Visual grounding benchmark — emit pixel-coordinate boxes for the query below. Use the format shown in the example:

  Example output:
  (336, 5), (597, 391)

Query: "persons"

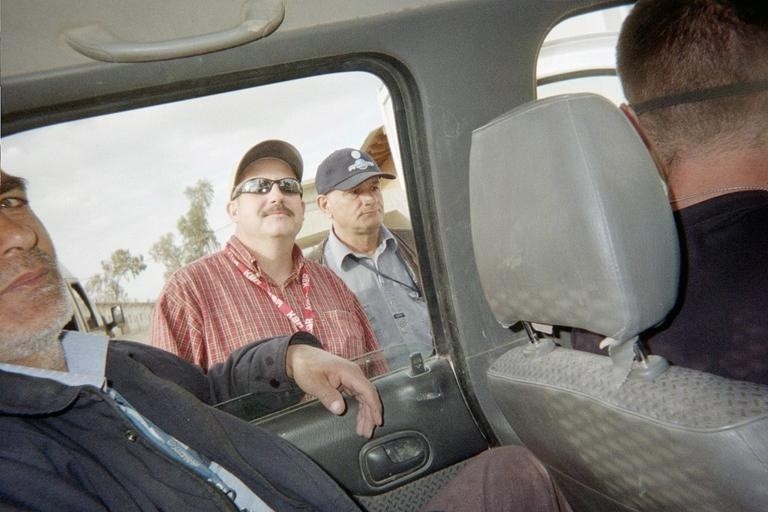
(145, 137), (392, 407)
(0, 171), (570, 512)
(305, 147), (437, 372)
(551, 2), (768, 386)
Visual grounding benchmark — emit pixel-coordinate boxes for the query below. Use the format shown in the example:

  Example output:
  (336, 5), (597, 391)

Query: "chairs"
(467, 92), (768, 503)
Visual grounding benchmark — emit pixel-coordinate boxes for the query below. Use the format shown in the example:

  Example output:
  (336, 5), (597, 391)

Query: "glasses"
(232, 176), (304, 202)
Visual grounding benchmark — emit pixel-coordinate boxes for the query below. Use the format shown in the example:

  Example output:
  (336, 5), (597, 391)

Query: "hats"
(315, 148), (396, 194)
(229, 138), (304, 188)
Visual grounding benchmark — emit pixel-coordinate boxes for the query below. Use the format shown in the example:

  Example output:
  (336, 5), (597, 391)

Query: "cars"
(57, 262), (126, 339)
